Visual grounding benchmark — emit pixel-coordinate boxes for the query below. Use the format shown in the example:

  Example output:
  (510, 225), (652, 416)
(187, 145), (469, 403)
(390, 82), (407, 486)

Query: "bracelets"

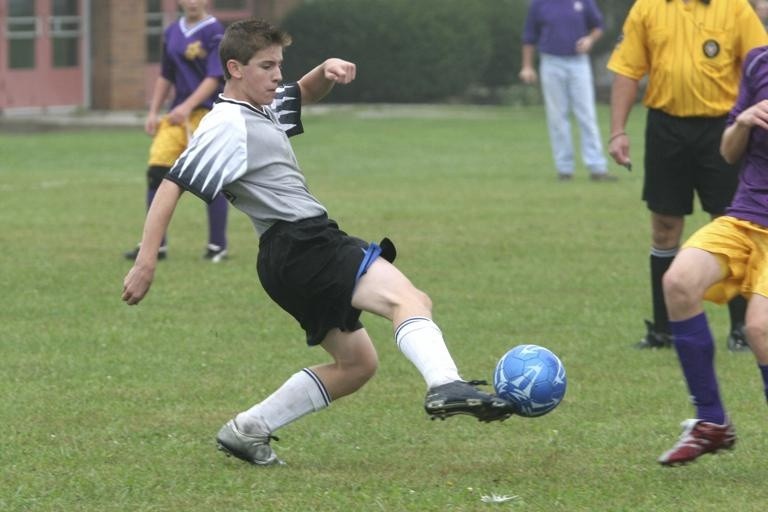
(609, 130), (629, 146)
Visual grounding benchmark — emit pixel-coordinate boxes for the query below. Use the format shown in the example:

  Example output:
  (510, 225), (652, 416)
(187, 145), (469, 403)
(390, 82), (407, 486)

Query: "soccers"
(495, 345), (567, 417)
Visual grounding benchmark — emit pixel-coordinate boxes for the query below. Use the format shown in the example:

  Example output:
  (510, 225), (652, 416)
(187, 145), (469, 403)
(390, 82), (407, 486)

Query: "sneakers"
(215, 421), (284, 466)
(424, 379), (512, 424)
(635, 314), (676, 349)
(724, 327), (746, 349)
(126, 242), (167, 260)
(657, 414), (736, 466)
(205, 244), (227, 260)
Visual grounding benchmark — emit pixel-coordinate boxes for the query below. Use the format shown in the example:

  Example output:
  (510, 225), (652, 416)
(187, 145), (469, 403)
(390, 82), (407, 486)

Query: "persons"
(123, 0), (230, 267)
(517, 0), (615, 181)
(117, 18), (517, 465)
(607, 0), (767, 355)
(655, 44), (768, 467)
